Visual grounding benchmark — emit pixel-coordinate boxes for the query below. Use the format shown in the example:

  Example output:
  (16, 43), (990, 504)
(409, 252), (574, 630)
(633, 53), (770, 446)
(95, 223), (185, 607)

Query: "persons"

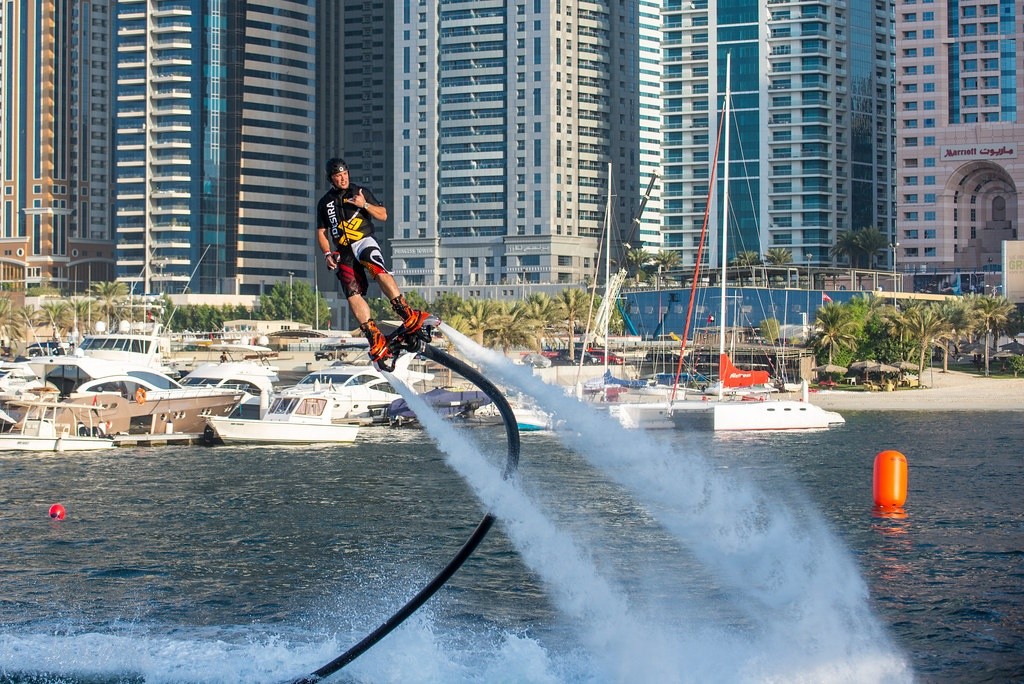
(220, 351), (227, 362)
(317, 157), (417, 357)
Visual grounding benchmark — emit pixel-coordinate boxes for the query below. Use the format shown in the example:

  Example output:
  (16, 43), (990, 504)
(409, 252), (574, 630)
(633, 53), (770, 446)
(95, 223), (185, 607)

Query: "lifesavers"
(135, 387), (147, 404)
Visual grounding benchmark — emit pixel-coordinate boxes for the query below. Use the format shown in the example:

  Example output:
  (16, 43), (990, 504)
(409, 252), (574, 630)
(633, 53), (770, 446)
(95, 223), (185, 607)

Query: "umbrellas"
(998, 339), (1024, 354)
(993, 350), (1019, 363)
(892, 361), (919, 378)
(865, 362), (899, 381)
(850, 359), (877, 381)
(811, 363), (848, 382)
(959, 339), (997, 362)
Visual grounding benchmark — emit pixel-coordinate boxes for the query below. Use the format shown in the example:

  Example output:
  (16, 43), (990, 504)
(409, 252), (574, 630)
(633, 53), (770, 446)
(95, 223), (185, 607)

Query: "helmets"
(326, 158), (349, 184)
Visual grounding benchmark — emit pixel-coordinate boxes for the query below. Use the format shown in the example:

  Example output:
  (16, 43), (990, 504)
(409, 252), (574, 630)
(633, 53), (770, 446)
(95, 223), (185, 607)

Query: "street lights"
(889, 242), (900, 308)
(805, 254), (813, 325)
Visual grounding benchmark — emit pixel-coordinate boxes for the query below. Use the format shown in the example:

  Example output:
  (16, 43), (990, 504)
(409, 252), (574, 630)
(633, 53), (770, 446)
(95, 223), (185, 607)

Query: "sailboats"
(473, 53), (846, 434)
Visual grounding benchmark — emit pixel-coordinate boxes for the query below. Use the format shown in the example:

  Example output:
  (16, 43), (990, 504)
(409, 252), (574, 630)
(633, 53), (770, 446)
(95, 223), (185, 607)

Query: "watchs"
(363, 202), (370, 208)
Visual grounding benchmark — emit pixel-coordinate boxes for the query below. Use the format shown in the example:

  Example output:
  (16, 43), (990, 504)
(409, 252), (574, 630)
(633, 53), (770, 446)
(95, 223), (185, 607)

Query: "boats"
(198, 392), (360, 445)
(0, 400), (116, 452)
(0, 242), (435, 445)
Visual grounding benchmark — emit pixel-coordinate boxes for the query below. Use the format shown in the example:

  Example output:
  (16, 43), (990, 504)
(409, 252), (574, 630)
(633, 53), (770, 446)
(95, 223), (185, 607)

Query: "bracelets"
(323, 249), (332, 259)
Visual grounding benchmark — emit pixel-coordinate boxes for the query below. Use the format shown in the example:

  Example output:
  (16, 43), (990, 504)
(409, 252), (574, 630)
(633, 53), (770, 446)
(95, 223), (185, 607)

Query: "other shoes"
(360, 319), (386, 356)
(390, 294), (420, 329)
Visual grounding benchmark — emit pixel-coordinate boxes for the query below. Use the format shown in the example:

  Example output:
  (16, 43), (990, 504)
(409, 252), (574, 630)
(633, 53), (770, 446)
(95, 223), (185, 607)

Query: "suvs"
(314, 344), (349, 362)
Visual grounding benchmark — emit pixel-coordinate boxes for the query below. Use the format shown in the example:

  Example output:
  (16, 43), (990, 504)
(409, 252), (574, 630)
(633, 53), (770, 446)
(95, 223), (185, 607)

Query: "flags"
(822, 291), (832, 302)
